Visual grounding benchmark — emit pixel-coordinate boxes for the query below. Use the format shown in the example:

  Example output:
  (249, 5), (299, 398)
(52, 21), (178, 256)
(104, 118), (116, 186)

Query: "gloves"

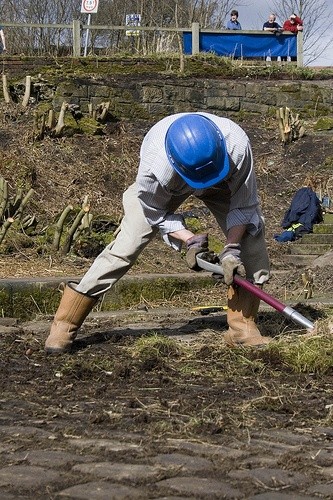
(217, 243), (247, 286)
(185, 233), (219, 271)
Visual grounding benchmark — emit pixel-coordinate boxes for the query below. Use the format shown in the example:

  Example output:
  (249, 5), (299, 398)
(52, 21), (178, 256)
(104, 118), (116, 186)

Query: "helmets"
(165, 114), (230, 189)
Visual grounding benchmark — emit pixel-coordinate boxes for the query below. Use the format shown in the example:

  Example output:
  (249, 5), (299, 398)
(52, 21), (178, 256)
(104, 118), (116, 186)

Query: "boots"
(45, 281), (100, 351)
(225, 283), (276, 348)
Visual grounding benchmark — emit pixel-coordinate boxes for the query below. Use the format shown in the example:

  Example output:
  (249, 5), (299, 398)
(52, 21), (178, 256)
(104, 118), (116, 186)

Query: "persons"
(45, 111), (276, 354)
(262, 14), (285, 31)
(283, 14), (304, 32)
(226, 10), (242, 30)
